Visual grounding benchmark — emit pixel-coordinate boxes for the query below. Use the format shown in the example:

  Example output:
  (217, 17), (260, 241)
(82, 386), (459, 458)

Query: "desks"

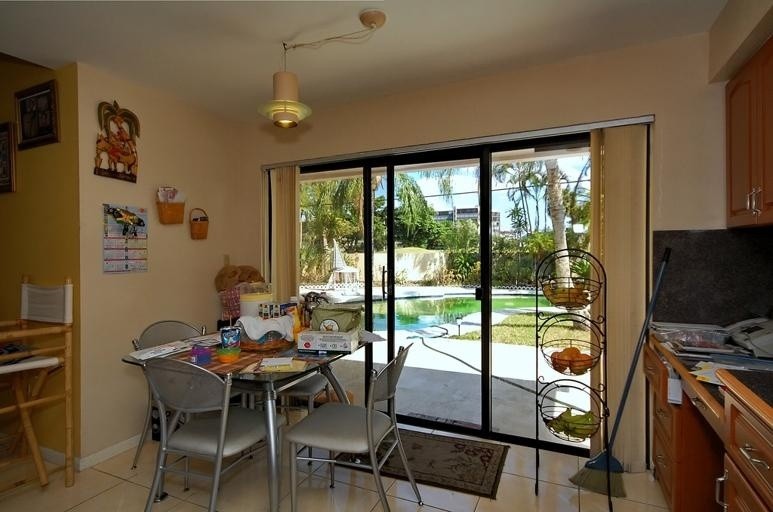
(643, 334), (773, 511)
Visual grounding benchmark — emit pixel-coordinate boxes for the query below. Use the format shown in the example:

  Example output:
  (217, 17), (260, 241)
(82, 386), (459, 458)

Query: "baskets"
(237, 323), (293, 351)
(540, 318), (601, 376)
(540, 385), (600, 443)
(540, 255), (602, 309)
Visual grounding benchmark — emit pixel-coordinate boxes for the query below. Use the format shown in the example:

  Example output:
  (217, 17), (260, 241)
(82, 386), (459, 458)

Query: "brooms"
(566, 248), (677, 498)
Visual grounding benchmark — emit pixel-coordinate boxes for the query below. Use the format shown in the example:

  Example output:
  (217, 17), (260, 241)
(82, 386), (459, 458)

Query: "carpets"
(328, 427), (511, 500)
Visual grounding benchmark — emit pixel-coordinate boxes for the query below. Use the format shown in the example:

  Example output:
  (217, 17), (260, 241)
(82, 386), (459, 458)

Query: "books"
(664, 337), (752, 386)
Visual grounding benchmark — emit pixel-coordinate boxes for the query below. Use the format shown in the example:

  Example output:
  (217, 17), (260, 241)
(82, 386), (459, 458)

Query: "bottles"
(258, 296), (297, 320)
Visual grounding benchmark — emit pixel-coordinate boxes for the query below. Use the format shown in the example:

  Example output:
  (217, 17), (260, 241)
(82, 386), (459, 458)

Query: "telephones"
(726, 318), (773, 350)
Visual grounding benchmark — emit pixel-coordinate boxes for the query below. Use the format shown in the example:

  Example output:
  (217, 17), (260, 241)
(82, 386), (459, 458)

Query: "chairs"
(284, 342), (424, 511)
(130, 320), (332, 511)
(0, 274), (75, 489)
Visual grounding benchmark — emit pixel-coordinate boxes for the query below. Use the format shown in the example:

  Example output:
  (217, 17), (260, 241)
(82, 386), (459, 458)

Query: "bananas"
(544, 280), (589, 307)
(547, 407), (594, 438)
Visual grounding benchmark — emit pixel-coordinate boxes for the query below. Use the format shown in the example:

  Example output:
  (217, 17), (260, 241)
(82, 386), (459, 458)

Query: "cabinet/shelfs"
(725, 35), (773, 230)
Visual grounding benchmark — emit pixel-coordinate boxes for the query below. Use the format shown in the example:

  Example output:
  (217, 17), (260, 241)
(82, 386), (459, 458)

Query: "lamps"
(256, 8), (386, 130)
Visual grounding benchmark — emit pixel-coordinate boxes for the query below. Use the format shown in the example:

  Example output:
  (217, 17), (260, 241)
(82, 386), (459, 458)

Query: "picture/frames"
(14, 78), (62, 152)
(0, 121), (16, 193)
